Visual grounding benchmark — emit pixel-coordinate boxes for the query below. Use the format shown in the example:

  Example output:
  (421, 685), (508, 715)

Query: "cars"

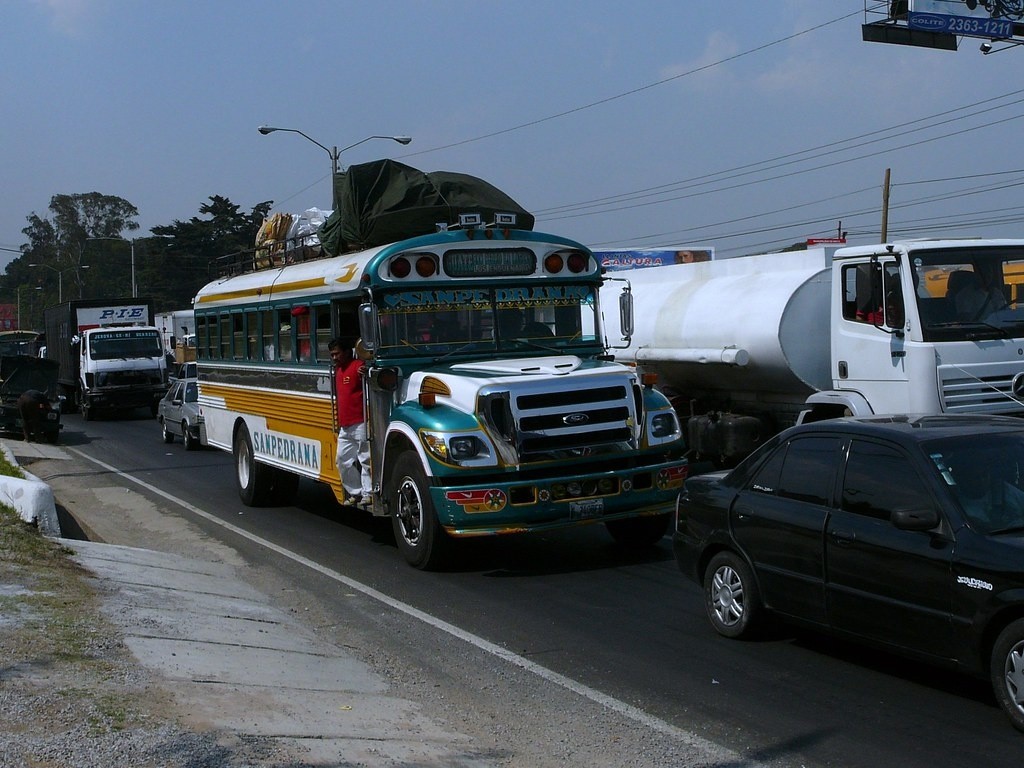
(675, 415), (1024, 724)
(158, 379), (199, 448)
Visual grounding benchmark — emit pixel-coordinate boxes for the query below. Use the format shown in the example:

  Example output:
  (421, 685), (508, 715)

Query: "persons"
(17, 389), (51, 443)
(857, 268), (919, 328)
(956, 260), (1009, 322)
(327, 337), (375, 506)
(82, 341), (99, 354)
(677, 250), (695, 263)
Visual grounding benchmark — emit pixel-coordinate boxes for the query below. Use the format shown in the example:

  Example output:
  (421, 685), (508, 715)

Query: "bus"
(0, 330), (40, 357)
(194, 229), (688, 568)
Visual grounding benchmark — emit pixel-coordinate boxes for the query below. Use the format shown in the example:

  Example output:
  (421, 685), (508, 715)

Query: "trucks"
(43, 298), (167, 420)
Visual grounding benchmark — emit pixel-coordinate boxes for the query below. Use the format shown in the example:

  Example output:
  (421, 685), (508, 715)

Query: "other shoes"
(344, 495), (363, 505)
(359, 497), (372, 506)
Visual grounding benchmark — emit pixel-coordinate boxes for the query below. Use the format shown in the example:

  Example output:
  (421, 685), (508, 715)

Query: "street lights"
(86, 235), (175, 298)
(258, 125), (412, 209)
(29, 264), (90, 303)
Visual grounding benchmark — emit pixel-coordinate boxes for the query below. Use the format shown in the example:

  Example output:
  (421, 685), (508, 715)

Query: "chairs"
(221, 320), (232, 359)
(263, 334), (275, 361)
(946, 271), (1007, 324)
(279, 330), (293, 361)
(316, 329), (332, 360)
(248, 335), (260, 361)
(234, 332), (246, 361)
(209, 324), (220, 358)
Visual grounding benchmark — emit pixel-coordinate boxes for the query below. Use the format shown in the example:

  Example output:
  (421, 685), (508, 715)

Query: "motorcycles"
(41, 395), (66, 444)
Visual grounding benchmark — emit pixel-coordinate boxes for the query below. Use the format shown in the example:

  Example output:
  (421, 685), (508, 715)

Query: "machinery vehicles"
(551, 239), (1024, 474)
